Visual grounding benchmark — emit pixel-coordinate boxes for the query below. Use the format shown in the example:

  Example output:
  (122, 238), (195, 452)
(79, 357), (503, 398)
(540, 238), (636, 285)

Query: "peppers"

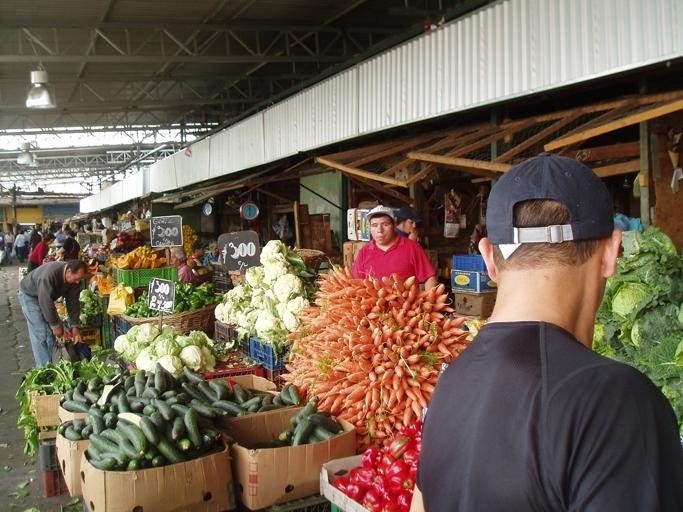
(122, 281), (222, 317)
(332, 420), (423, 512)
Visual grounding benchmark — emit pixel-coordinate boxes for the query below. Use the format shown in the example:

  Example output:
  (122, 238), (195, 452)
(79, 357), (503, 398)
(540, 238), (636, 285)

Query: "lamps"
(24, 59), (57, 110)
(16, 139), (41, 169)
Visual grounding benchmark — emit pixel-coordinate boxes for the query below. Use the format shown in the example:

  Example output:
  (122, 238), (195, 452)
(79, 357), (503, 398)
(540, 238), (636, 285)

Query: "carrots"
(276, 255), (472, 453)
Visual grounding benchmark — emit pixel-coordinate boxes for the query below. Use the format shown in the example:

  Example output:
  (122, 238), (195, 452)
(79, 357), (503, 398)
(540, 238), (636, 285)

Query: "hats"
(191, 240), (209, 251)
(396, 206), (423, 223)
(366, 205), (395, 223)
(485, 152), (614, 260)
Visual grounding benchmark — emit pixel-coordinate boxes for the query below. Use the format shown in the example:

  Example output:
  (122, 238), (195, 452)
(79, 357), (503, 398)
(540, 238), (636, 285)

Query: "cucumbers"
(59, 362), (343, 471)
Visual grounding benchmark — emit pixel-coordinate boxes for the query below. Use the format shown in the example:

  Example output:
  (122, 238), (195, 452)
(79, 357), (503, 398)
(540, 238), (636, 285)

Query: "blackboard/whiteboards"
(218, 230), (260, 271)
(148, 278), (175, 314)
(149, 214), (183, 247)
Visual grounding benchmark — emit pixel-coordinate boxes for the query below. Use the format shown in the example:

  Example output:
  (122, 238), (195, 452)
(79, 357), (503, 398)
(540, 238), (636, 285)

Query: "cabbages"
(79, 289), (101, 323)
(591, 222), (683, 436)
(113, 322), (217, 380)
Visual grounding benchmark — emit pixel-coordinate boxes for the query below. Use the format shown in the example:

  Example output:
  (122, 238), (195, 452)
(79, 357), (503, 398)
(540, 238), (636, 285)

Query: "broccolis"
(213, 239), (310, 343)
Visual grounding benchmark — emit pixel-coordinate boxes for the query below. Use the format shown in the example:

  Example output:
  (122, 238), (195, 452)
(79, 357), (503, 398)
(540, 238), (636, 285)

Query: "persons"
(185, 240), (209, 269)
(0, 217), (105, 263)
(349, 204), (438, 291)
(394, 206), (420, 238)
(170, 248), (194, 283)
(17, 260), (88, 368)
(407, 149), (682, 512)
(203, 240), (220, 267)
(27, 233), (56, 272)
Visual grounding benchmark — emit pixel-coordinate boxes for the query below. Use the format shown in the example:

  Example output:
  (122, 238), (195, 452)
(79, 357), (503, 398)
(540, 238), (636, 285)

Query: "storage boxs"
(34, 392), (66, 438)
(39, 440), (72, 498)
(211, 373), (275, 403)
(78, 431), (234, 510)
(53, 415), (90, 499)
(234, 388), (300, 420)
(56, 400), (90, 424)
(450, 268), (497, 321)
(234, 405), (357, 511)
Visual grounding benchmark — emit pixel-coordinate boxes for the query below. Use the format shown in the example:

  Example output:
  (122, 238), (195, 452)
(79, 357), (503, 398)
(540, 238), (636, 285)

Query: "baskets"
(112, 263), (178, 288)
(38, 438), (70, 498)
(292, 249), (325, 278)
(114, 302), (220, 339)
(452, 254), (488, 273)
(210, 264), (234, 297)
(264, 492), (345, 512)
(192, 320), (290, 392)
(63, 324), (101, 346)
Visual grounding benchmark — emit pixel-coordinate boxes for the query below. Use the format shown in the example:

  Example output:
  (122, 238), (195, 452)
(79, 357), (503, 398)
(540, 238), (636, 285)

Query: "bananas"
(116, 246), (163, 269)
